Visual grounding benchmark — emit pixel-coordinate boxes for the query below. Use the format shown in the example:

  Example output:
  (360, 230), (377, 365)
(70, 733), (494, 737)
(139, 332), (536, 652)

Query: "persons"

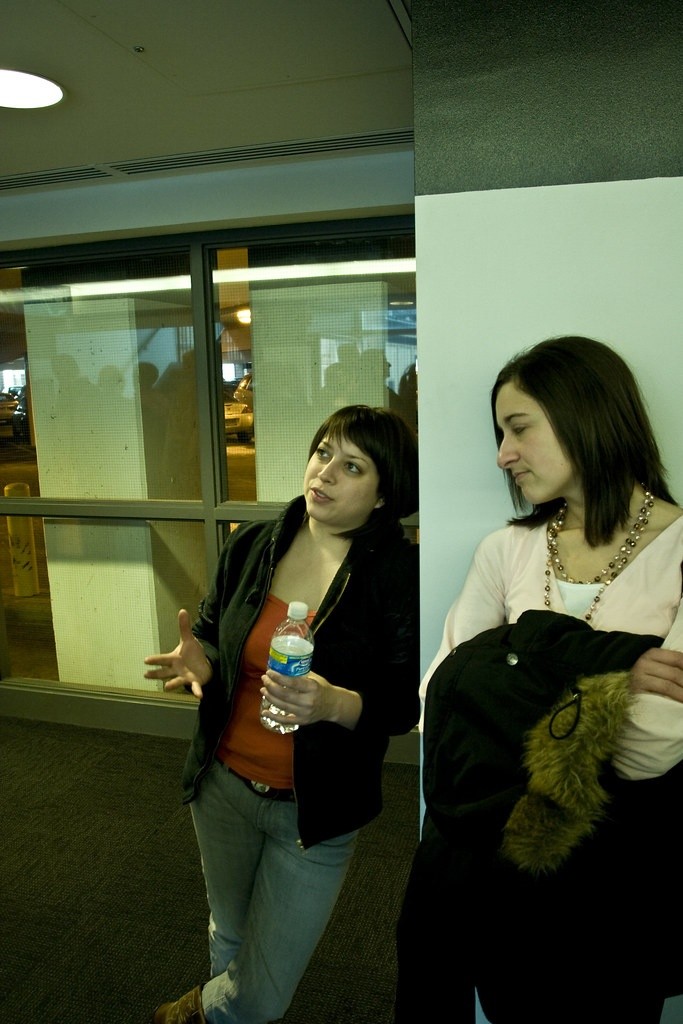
(37, 349), (198, 488)
(143, 405), (420, 1024)
(393, 336), (683, 1024)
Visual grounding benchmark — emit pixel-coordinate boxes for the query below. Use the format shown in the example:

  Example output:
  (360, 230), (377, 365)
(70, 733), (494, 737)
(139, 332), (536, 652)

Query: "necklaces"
(544, 483), (654, 620)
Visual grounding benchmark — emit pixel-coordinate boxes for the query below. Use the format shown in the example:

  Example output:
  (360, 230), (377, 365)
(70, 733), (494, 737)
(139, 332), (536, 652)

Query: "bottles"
(259, 600), (316, 735)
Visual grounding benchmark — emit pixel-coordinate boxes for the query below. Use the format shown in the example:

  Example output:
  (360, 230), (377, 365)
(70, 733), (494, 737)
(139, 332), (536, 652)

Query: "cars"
(10, 385), (29, 442)
(233, 375), (253, 411)
(9, 386), (23, 397)
(224, 392), (254, 443)
(0, 393), (19, 424)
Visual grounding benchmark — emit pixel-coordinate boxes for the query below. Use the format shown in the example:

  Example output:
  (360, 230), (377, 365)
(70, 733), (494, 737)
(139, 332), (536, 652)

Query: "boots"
(155, 983), (206, 1024)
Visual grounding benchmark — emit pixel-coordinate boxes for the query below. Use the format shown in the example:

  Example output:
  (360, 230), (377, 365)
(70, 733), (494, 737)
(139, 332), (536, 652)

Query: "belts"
(214, 753), (296, 803)
